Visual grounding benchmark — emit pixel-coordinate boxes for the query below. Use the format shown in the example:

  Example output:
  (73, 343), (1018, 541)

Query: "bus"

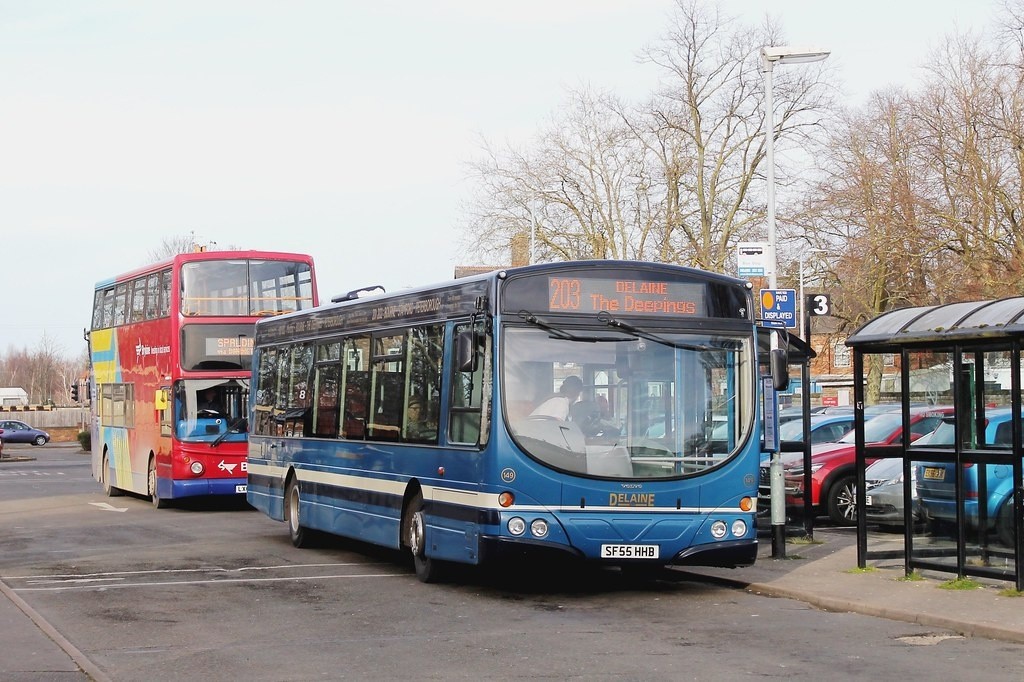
(245, 258), (789, 585)
(83, 252), (320, 510)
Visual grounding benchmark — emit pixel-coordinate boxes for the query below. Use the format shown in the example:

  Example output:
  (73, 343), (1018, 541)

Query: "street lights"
(798, 248), (830, 418)
(530, 189), (559, 264)
(760, 44), (831, 563)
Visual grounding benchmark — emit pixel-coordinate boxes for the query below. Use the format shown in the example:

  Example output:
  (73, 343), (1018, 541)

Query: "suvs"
(759, 405), (955, 527)
(916, 404), (1024, 546)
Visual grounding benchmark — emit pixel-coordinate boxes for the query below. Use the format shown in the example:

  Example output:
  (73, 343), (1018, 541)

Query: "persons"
(406, 395), (438, 439)
(528, 376), (584, 422)
(197, 387), (222, 418)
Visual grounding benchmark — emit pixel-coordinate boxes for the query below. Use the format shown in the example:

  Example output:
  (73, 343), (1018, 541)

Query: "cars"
(0, 420), (51, 446)
(621, 402), (918, 549)
(864, 429), (935, 533)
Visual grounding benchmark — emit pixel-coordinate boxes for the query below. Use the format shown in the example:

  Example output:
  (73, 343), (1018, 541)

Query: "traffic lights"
(70, 384), (79, 401)
